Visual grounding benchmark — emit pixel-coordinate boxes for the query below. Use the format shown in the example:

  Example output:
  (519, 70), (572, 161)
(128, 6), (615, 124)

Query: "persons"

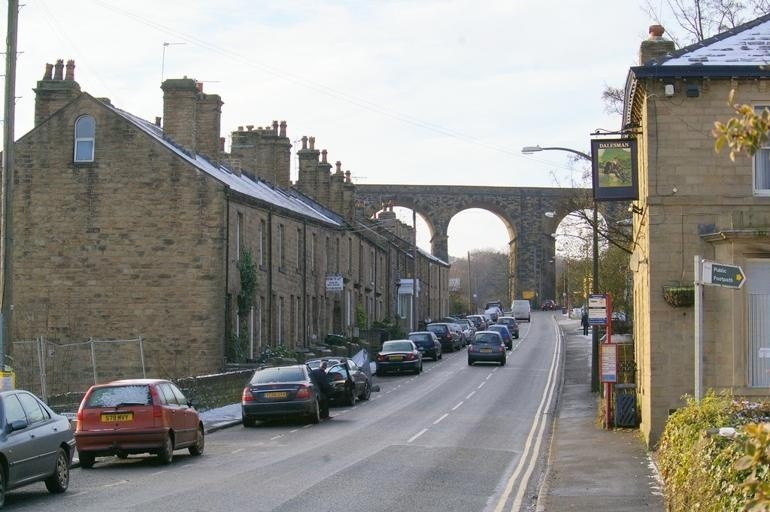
(312, 362), (330, 418)
(581, 312), (589, 336)
(600, 155), (628, 183)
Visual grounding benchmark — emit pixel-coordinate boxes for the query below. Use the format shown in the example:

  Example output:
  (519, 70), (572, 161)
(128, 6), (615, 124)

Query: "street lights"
(473, 294), (477, 315)
(522, 144), (601, 394)
(550, 232), (591, 318)
(551, 256), (571, 319)
(543, 209), (594, 225)
(548, 260), (567, 315)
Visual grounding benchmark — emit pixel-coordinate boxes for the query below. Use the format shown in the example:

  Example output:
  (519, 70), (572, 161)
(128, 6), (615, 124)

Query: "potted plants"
(663, 284), (696, 307)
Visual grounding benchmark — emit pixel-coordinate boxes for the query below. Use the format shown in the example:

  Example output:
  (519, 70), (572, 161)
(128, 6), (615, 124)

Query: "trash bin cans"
(614, 383), (637, 429)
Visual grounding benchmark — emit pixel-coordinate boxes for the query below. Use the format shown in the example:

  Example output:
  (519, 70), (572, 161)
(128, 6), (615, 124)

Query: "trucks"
(511, 299), (531, 324)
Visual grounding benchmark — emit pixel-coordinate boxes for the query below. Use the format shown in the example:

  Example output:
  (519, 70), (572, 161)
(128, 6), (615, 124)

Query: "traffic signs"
(702, 258), (747, 290)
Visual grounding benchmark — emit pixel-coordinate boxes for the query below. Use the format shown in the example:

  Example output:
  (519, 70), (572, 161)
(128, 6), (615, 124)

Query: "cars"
(73, 379), (204, 466)
(497, 316), (519, 339)
(1, 389), (77, 510)
(302, 356), (371, 406)
(371, 339), (424, 376)
(540, 298), (557, 312)
(241, 362), (329, 428)
(468, 331), (509, 366)
(407, 300), (504, 361)
(486, 324), (512, 350)
